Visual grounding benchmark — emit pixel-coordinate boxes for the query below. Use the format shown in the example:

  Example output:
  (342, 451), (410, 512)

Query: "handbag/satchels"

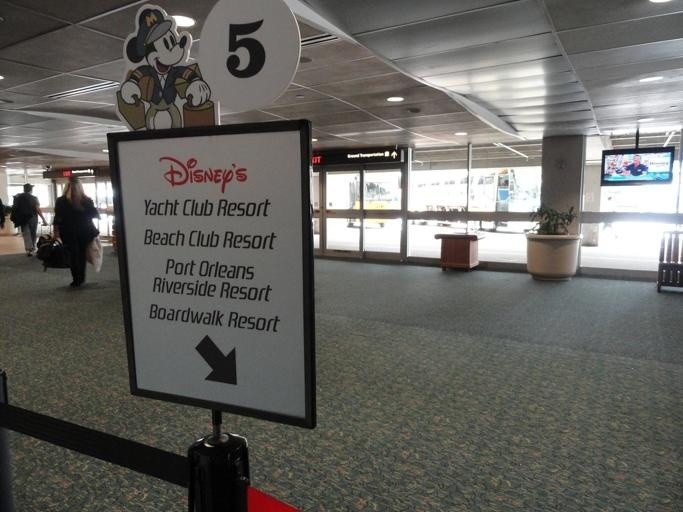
(85, 235), (103, 272)
(42, 239), (70, 268)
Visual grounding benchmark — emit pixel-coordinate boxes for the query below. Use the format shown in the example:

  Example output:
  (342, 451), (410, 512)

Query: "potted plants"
(523, 203), (583, 280)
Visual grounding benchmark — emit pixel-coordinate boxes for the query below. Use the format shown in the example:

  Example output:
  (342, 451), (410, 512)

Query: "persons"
(622, 154), (648, 176)
(49, 178), (100, 288)
(10, 184), (48, 256)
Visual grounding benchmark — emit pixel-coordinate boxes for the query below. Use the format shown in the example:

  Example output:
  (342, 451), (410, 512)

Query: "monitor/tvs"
(600, 146), (674, 186)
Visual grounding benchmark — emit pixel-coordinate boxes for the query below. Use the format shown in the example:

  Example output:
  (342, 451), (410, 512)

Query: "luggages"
(37, 224), (53, 261)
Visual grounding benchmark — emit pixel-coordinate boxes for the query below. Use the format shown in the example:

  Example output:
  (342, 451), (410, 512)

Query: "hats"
(24, 184), (34, 191)
